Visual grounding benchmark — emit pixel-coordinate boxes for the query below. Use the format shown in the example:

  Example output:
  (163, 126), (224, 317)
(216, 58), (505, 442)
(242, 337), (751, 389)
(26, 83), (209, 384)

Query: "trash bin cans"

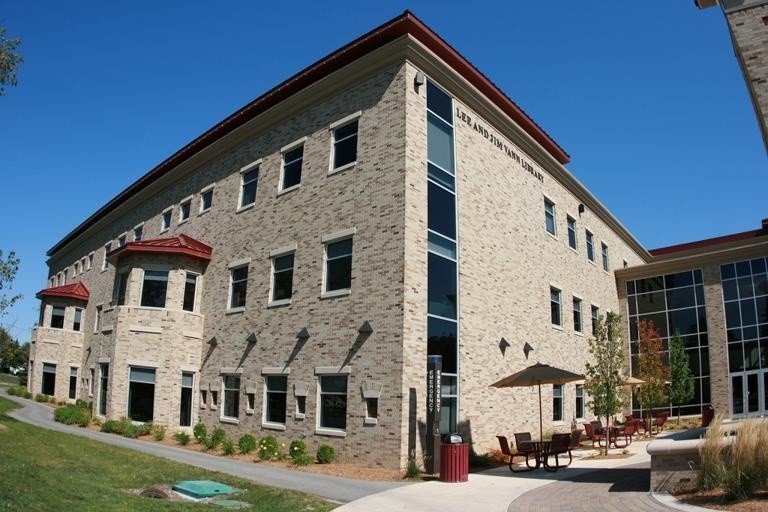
(438, 433), (470, 482)
(701, 404), (713, 427)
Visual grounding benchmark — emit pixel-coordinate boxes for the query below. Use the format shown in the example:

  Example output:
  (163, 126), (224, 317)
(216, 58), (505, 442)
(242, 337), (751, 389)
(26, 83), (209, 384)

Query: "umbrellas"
(487, 360), (585, 462)
(636, 375), (672, 390)
(576, 373), (647, 440)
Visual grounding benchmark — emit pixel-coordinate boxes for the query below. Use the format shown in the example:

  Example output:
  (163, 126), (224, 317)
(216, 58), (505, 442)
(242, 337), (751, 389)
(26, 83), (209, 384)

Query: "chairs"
(496, 412), (669, 473)
